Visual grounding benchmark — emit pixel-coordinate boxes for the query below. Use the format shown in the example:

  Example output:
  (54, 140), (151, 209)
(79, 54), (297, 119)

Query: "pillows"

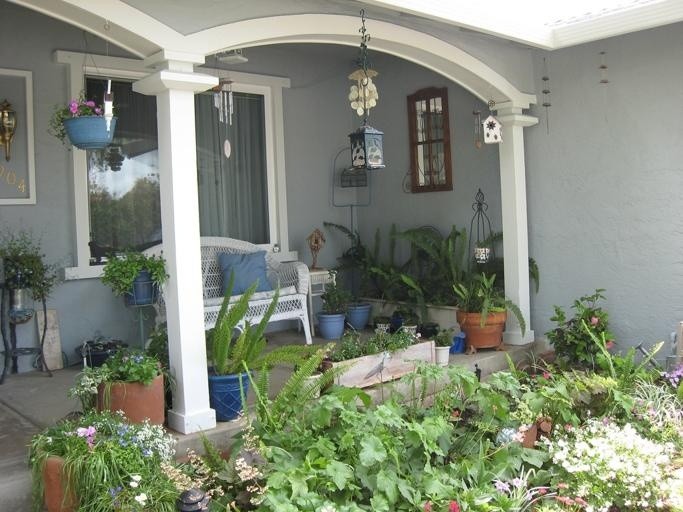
(215, 250), (273, 298)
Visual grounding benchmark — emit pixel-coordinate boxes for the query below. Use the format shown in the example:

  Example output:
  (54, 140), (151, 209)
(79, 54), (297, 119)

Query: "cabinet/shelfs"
(0, 277), (53, 384)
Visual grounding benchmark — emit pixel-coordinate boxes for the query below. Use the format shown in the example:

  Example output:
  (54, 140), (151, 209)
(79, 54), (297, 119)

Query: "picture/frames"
(0, 68), (37, 206)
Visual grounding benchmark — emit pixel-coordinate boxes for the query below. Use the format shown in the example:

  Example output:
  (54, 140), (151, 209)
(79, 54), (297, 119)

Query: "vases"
(62, 116), (119, 150)
(311, 249), (321, 270)
(97, 374), (165, 425)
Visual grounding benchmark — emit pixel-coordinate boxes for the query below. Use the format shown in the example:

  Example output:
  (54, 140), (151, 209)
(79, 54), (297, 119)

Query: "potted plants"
(102, 251), (165, 305)
(0, 222), (61, 315)
(315, 212), (540, 353)
(205, 267), (683, 512)
(473, 231), (502, 264)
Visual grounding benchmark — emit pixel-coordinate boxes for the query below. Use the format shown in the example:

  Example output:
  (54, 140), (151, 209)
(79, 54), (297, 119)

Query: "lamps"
(348, 120), (386, 171)
(0, 97), (18, 163)
(344, 9), (379, 117)
(219, 49), (248, 65)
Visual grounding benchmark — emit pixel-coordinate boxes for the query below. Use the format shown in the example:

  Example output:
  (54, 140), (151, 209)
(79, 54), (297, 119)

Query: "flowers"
(26, 408), (212, 512)
(67, 348), (178, 411)
(306, 229), (325, 250)
(45, 88), (105, 145)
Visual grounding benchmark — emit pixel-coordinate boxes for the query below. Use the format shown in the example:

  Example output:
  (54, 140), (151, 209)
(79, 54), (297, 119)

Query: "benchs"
(135, 235), (315, 354)
